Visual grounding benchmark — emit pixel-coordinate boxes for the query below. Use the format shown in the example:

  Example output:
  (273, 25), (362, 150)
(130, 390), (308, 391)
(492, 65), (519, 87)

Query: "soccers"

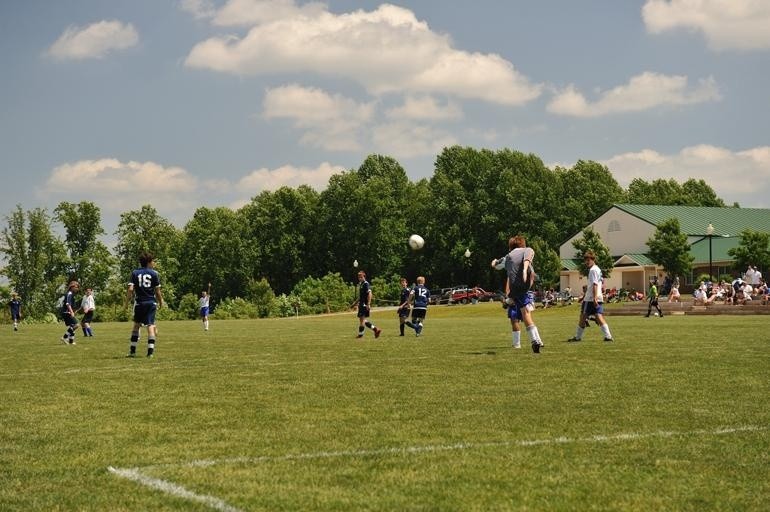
(410, 235), (425, 249)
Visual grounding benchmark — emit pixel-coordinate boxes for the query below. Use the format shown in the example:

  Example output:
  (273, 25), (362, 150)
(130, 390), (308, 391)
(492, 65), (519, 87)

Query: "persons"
(74, 287), (95, 337)
(197, 281), (212, 331)
(123, 253), (163, 359)
(490, 234), (770, 358)
(6, 293), (21, 331)
(60, 281), (80, 345)
(397, 278), (419, 336)
(409, 276), (432, 337)
(350, 271), (381, 339)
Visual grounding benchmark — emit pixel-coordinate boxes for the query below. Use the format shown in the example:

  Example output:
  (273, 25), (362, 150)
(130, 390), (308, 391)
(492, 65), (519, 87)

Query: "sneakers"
(568, 337), (582, 342)
(601, 337), (613, 344)
(125, 351), (136, 358)
(511, 339), (544, 353)
(356, 335), (363, 338)
(416, 323), (423, 337)
(145, 353), (153, 359)
(60, 336), (77, 345)
(375, 328), (381, 338)
(205, 328), (208, 331)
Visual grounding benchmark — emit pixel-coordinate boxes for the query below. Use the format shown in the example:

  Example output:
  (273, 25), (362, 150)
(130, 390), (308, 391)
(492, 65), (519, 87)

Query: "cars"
(428, 285), (504, 304)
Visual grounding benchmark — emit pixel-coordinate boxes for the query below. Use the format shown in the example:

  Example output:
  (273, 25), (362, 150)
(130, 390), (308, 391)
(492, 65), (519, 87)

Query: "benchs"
(711, 283), (770, 306)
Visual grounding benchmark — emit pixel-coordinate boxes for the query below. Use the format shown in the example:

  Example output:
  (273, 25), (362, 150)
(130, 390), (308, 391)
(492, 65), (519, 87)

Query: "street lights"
(706, 221), (717, 280)
